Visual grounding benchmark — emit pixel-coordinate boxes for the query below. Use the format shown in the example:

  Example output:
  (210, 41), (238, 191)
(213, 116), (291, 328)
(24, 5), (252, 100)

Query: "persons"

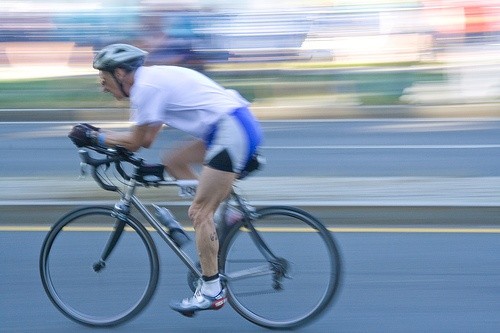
(67, 43), (260, 311)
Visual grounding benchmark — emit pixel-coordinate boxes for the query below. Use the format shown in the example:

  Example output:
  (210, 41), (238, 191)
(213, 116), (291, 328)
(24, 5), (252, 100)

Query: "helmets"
(92, 43), (148, 70)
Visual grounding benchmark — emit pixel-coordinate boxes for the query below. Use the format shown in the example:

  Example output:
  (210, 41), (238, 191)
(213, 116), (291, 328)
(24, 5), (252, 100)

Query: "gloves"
(68, 123), (101, 148)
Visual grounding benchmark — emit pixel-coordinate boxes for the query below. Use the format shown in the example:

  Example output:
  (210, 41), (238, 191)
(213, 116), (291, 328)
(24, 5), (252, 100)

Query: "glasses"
(97, 74), (117, 87)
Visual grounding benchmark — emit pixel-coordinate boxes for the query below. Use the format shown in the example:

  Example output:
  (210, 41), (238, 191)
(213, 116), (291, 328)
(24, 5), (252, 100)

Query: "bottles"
(151, 204), (189, 246)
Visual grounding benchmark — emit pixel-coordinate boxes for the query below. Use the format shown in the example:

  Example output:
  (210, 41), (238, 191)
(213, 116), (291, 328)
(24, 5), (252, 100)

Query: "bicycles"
(39, 124), (342, 331)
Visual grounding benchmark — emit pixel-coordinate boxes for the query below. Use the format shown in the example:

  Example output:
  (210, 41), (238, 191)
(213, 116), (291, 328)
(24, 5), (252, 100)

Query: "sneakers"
(213, 197), (255, 229)
(168, 288), (227, 312)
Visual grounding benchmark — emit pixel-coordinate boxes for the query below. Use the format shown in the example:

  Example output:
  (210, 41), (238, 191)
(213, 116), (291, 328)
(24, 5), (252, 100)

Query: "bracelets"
(97, 133), (105, 147)
